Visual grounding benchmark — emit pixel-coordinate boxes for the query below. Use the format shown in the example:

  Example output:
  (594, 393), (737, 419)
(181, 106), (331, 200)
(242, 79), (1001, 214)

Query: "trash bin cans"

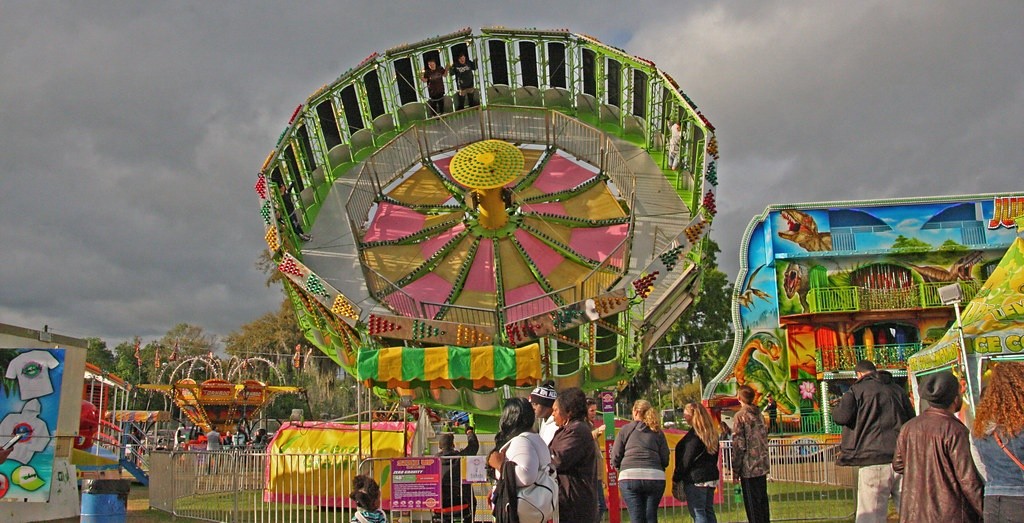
(80, 478), (128, 523)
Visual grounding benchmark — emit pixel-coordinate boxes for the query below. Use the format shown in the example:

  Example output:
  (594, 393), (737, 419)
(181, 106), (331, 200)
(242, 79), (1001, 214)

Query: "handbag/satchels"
(614, 444), (626, 470)
(672, 478), (687, 502)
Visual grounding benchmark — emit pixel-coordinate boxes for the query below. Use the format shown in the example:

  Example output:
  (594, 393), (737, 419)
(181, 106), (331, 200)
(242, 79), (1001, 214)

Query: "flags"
(292, 344), (314, 369)
(133, 342), (180, 371)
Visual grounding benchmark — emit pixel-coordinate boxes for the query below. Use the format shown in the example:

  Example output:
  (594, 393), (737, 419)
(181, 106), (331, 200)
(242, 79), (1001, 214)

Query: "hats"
(918, 371), (958, 407)
(531, 380), (558, 406)
(439, 434), (454, 449)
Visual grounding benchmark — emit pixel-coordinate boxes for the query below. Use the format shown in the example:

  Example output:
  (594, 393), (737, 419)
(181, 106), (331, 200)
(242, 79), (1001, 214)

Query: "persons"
(428, 424), (479, 523)
(764, 393), (777, 434)
(890, 370), (985, 523)
(207, 424), (267, 475)
(276, 182), (312, 242)
(731, 385), (771, 523)
(486, 380), (607, 523)
(970, 361), (1024, 523)
(832, 360), (917, 523)
(672, 402), (720, 523)
(611, 400), (670, 523)
(129, 427), (140, 463)
(349, 475), (389, 523)
(718, 421), (731, 441)
(663, 117), (687, 170)
(418, 54), (478, 119)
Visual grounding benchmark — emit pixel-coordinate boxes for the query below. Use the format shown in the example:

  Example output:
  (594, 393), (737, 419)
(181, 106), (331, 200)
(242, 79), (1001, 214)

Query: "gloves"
(464, 423), (473, 433)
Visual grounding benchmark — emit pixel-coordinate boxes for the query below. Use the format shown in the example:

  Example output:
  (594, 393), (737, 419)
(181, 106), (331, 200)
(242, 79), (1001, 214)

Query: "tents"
(906, 236), (1024, 431)
(356, 342), (539, 460)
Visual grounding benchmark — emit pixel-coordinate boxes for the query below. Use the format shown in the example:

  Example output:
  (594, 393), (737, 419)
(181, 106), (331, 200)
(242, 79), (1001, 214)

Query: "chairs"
(232, 435), (246, 449)
(252, 435), (267, 449)
